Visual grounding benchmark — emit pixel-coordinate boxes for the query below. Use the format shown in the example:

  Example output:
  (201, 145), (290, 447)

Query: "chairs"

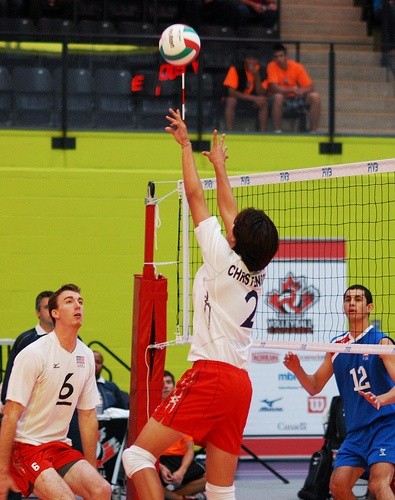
(0, 0), (394, 134)
(322, 396), (372, 500)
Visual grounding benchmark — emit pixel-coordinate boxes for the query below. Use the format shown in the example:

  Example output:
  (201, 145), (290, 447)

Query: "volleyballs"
(159, 23), (201, 66)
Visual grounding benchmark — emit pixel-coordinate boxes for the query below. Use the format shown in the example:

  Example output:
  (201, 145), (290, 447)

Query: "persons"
(121, 109), (280, 500)
(0, 0), (395, 56)
(266, 42), (321, 133)
(222, 46), (268, 132)
(66, 350), (129, 489)
(157, 370), (208, 500)
(0, 291), (54, 415)
(0, 283), (113, 500)
(282, 284), (395, 500)
